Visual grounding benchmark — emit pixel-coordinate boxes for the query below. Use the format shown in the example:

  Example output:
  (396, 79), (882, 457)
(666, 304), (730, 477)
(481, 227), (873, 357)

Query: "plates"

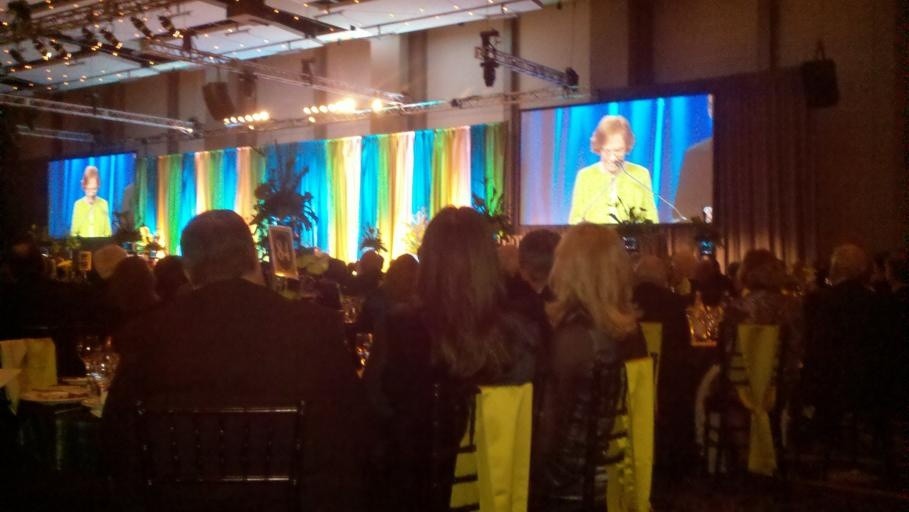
(21, 389), (92, 405)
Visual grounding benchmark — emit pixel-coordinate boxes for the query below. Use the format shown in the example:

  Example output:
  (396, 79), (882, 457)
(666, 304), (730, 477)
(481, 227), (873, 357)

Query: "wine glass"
(78, 336), (103, 369)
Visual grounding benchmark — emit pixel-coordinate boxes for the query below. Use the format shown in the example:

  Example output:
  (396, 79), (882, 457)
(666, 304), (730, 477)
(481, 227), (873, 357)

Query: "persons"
(566, 115), (660, 225)
(114, 153), (137, 235)
(69, 166), (112, 238)
(672, 95), (716, 227)
(667, 238), (909, 508)
(0, 207), (698, 510)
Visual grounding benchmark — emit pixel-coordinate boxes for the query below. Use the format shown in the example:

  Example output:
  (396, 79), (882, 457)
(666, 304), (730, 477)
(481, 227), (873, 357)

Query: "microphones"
(609, 154), (687, 220)
(92, 195), (118, 228)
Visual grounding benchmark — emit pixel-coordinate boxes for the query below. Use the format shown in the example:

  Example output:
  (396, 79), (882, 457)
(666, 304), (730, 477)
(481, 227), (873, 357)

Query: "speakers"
(201, 81), (235, 121)
(801, 59), (840, 108)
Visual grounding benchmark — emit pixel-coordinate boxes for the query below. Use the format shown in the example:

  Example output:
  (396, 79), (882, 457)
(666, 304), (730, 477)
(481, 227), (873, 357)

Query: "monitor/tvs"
(45, 150), (137, 240)
(517, 89), (719, 229)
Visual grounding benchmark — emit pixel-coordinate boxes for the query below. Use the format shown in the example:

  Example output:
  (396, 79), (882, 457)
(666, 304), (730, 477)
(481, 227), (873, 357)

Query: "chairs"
(131, 317), (798, 512)
